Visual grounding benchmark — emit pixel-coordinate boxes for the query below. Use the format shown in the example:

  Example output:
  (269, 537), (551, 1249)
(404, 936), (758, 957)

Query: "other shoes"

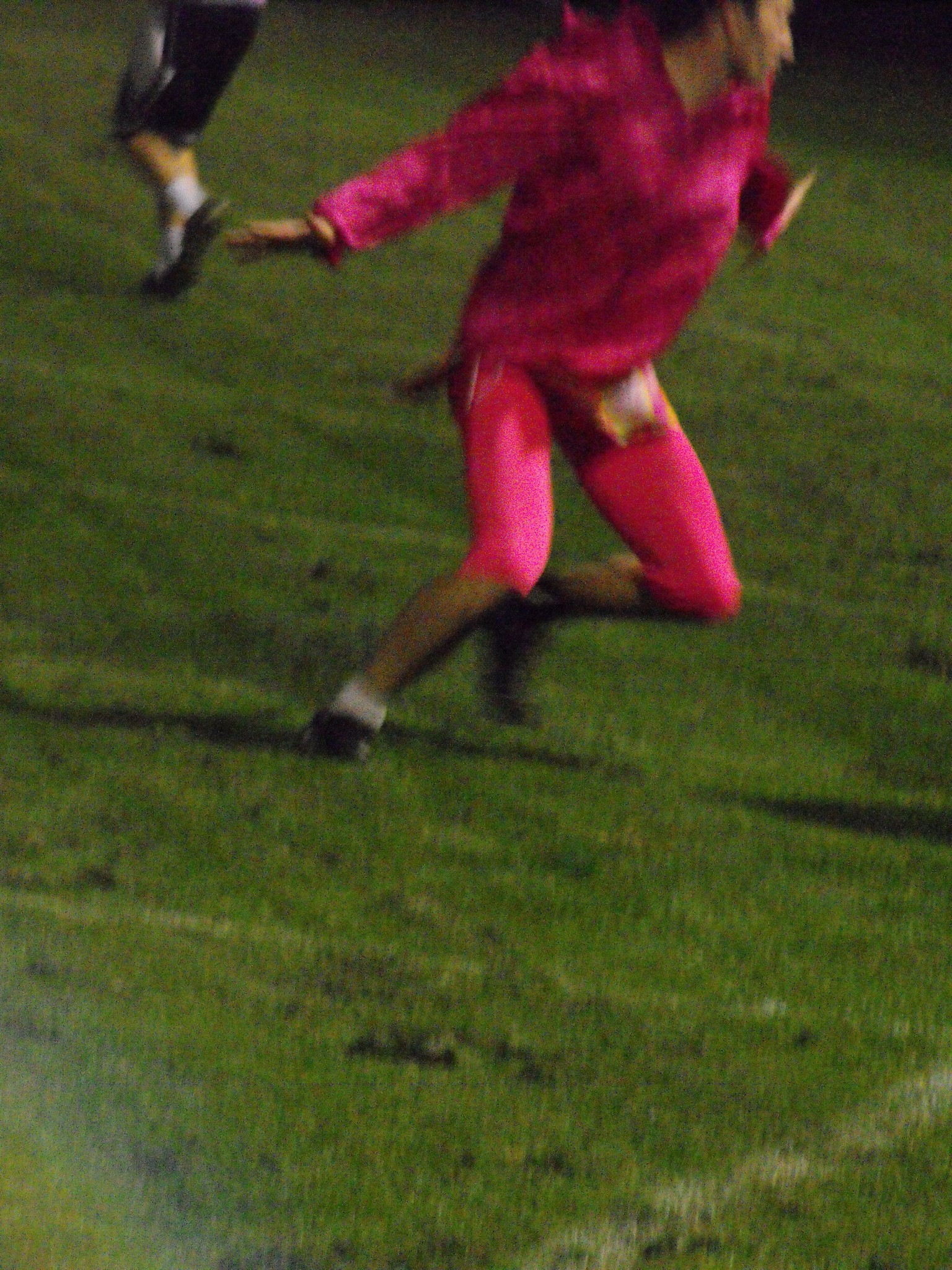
(141, 193), (228, 296)
(300, 705), (375, 770)
(481, 576), (559, 725)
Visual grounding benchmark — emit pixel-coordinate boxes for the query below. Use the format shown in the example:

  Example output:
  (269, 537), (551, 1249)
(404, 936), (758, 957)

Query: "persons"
(222, 0), (820, 762)
(112, 0), (267, 296)
